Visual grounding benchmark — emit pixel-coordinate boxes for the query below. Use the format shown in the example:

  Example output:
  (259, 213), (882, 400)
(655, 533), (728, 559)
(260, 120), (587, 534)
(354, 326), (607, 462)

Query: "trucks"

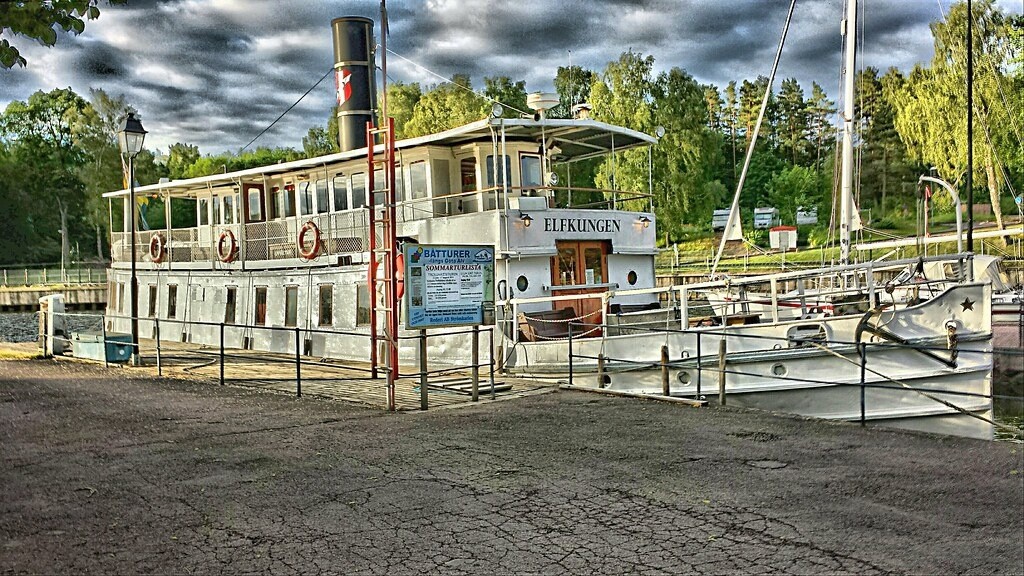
(712, 208), (731, 232)
(753, 206), (781, 229)
(797, 202), (819, 227)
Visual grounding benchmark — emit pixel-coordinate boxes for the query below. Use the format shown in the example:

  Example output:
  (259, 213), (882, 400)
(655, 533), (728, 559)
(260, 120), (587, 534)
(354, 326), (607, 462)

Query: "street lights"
(115, 111), (148, 367)
(931, 166), (936, 229)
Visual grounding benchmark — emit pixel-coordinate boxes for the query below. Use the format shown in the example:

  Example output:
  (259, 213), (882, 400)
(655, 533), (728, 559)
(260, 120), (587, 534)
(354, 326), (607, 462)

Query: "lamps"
(518, 210), (533, 227)
(654, 125), (665, 140)
(637, 215), (652, 228)
(545, 172), (558, 186)
(488, 103), (503, 118)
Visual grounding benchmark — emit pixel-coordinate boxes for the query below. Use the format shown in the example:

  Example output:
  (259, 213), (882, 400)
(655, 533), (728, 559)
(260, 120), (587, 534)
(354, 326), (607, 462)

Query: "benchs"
(268, 236), (360, 258)
(518, 306), (585, 342)
(611, 302), (661, 314)
(689, 313), (763, 327)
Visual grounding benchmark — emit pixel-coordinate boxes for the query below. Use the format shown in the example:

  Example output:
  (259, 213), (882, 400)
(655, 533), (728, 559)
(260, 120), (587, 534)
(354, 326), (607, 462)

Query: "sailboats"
(492, 0), (1000, 443)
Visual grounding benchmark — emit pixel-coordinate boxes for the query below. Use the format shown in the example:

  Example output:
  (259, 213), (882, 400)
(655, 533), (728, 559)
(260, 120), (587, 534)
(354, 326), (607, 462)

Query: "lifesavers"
(298, 224), (322, 261)
(366, 249), (407, 303)
(148, 234), (165, 262)
(217, 230), (236, 262)
